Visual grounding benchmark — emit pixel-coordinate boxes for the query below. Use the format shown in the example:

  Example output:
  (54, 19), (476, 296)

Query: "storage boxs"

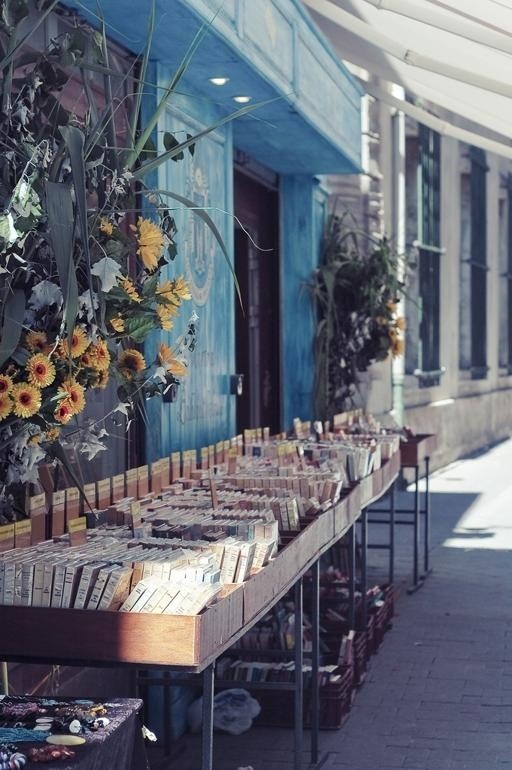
(213, 578), (395, 732)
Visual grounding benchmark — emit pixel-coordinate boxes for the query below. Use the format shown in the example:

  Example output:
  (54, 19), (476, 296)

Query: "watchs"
(60, 719), (99, 735)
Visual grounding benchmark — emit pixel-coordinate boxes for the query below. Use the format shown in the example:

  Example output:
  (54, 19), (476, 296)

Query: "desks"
(2, 694), (147, 768)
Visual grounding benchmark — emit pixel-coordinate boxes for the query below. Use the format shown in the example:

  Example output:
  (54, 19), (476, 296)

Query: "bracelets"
(28, 746), (76, 761)
(0, 751), (26, 770)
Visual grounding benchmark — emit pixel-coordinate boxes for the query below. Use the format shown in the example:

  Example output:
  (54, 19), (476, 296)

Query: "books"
(1, 507), (279, 614)
(210, 602), (341, 683)
(149, 470), (342, 532)
(286, 559), (388, 625)
(226, 435), (401, 488)
(323, 412), (418, 440)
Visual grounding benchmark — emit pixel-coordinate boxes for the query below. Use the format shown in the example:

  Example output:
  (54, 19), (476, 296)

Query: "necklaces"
(1, 710), (103, 732)
(0, 704), (107, 715)
(3, 697), (95, 707)
(0, 717), (58, 728)
(0, 728), (88, 745)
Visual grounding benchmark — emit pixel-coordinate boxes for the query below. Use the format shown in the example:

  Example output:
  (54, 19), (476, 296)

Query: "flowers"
(309, 196), (405, 418)
(1, 101), (251, 526)
